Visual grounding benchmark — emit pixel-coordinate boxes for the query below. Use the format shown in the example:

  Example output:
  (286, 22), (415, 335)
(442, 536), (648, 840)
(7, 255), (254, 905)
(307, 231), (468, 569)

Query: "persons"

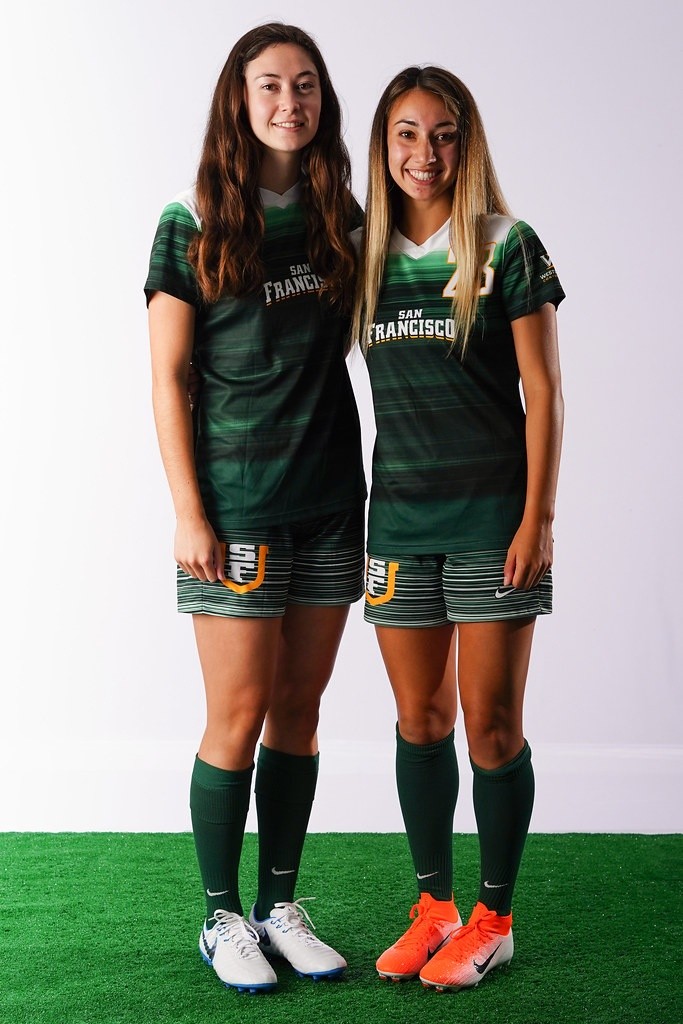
(351, 64), (565, 992)
(142, 21), (369, 990)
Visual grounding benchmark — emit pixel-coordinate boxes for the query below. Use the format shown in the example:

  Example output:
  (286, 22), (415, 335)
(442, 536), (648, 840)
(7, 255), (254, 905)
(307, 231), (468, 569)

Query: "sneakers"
(199, 910), (278, 995)
(374, 905), (462, 982)
(247, 900), (350, 981)
(418, 920), (515, 993)
